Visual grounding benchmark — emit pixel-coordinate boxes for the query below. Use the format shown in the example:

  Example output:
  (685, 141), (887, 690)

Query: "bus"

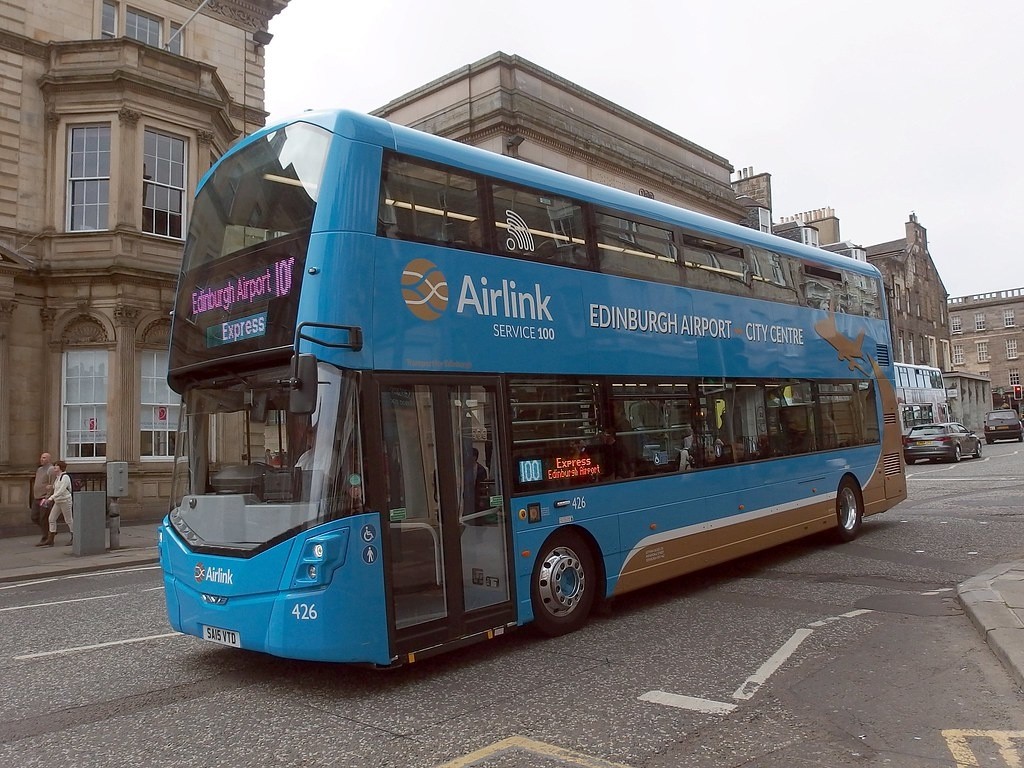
(894, 360), (952, 447)
(155, 108), (911, 673)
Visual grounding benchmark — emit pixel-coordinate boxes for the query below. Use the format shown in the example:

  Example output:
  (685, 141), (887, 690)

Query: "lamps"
(507, 136), (525, 148)
(253, 31), (274, 48)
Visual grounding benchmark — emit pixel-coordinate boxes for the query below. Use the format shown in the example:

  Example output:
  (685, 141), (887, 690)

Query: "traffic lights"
(1013, 385), (1022, 401)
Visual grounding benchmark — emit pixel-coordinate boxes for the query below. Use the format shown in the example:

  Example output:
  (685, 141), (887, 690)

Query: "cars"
(903, 422), (983, 465)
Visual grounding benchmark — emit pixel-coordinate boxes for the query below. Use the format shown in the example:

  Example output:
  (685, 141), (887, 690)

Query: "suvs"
(982, 408), (1024, 444)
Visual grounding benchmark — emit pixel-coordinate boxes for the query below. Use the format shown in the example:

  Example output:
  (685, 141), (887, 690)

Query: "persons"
(469, 219), (484, 247)
(704, 446), (716, 464)
(30, 453), (73, 547)
(293, 425), (339, 487)
(264, 448), (288, 466)
(734, 442), (746, 460)
(468, 449), (487, 496)
